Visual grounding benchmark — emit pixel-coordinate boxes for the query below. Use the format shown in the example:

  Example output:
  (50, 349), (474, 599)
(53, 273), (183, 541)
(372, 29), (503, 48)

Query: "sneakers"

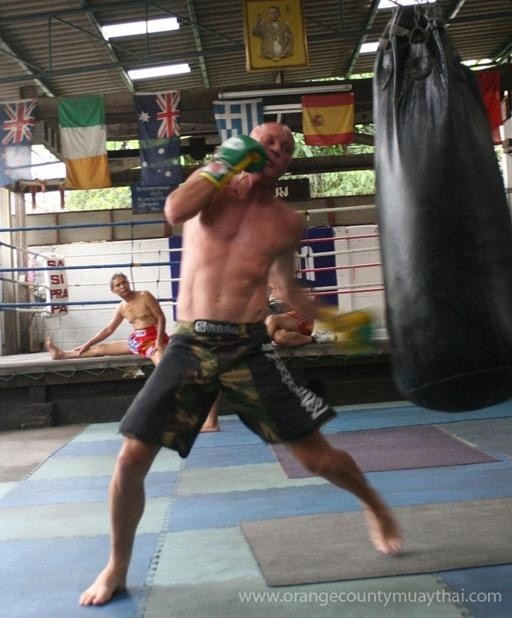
(312, 332), (337, 344)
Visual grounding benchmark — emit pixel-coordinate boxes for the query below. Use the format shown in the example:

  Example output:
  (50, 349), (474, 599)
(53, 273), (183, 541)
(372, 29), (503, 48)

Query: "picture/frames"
(242, 0), (309, 73)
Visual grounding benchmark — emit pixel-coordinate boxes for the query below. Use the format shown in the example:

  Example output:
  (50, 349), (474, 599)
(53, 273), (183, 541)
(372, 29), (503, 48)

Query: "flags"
(1, 99), (36, 185)
(131, 91), (184, 189)
(472, 59), (511, 144)
(56, 96), (113, 189)
(303, 92), (355, 147)
(211, 99), (266, 142)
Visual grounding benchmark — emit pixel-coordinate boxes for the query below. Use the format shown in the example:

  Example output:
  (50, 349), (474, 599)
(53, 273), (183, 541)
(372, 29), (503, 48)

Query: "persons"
(252, 5), (294, 62)
(44, 272), (168, 371)
(196, 390), (222, 433)
(260, 303), (339, 344)
(72, 114), (420, 613)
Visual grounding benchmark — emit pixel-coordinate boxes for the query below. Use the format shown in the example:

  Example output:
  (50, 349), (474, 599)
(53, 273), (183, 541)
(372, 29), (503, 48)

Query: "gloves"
(198, 134), (269, 194)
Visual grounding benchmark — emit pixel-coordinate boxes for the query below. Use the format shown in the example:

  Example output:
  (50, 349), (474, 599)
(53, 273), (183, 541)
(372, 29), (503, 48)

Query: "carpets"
(271, 423), (501, 480)
(240, 497), (512, 587)
(0, 423), (90, 483)
